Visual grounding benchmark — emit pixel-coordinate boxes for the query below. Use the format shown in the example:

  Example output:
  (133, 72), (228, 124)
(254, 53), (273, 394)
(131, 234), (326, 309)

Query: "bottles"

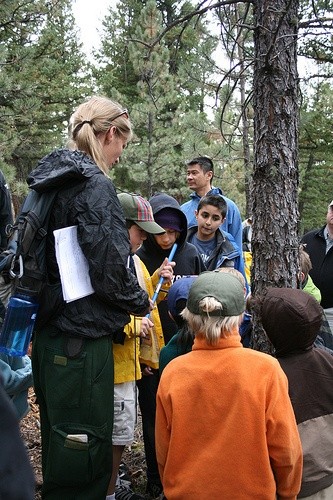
(0, 287), (39, 357)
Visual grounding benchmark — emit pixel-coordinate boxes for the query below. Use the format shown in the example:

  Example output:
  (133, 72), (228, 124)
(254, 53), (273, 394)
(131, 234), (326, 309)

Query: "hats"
(118, 193), (164, 236)
(155, 209), (183, 231)
(185, 269), (246, 316)
(167, 278), (197, 315)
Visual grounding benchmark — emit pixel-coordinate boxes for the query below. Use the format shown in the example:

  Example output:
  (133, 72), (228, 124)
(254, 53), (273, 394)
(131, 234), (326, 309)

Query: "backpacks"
(0, 189), (57, 334)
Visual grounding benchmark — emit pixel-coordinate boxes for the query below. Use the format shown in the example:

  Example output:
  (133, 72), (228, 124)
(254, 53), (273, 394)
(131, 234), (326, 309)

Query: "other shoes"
(114, 485), (139, 500)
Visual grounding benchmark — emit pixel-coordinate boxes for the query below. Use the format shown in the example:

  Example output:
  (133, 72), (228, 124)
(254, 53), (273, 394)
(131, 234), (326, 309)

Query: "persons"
(138, 193), (209, 500)
(154, 268), (303, 500)
(258, 286), (333, 500)
(104, 192), (176, 500)
(156, 156), (333, 372)
(1, 169), (15, 328)
(2, 94), (158, 500)
